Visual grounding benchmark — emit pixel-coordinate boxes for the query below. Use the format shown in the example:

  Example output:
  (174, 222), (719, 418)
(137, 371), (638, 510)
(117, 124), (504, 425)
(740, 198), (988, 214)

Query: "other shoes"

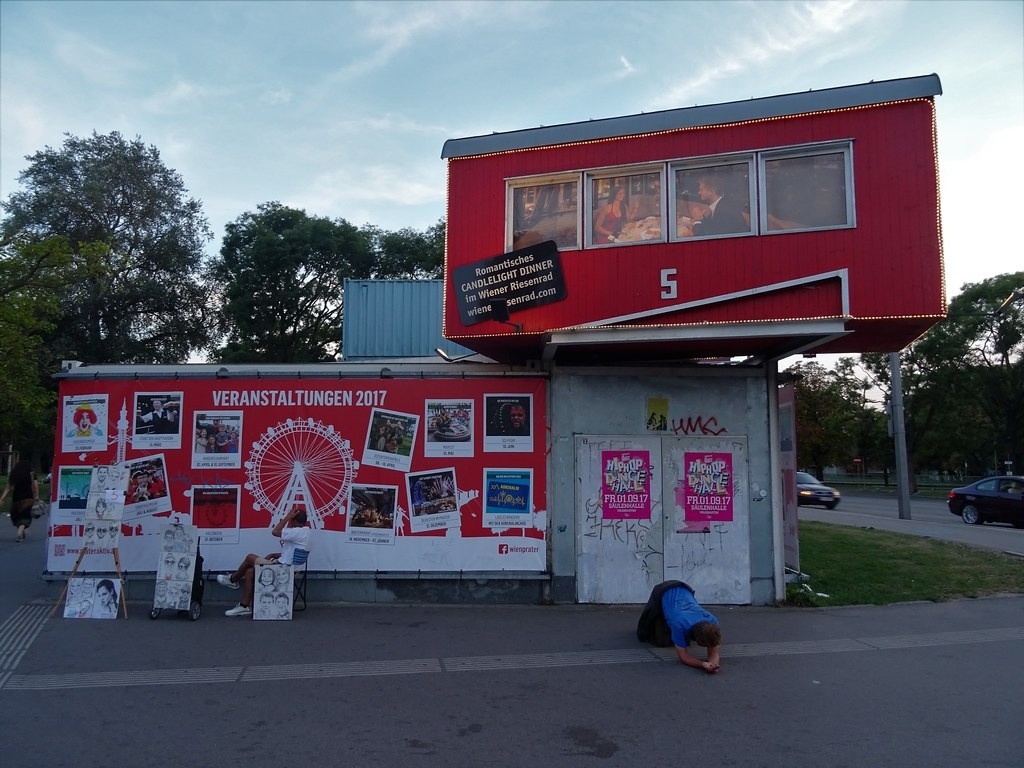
(16, 534), (24, 542)
(24, 531), (28, 538)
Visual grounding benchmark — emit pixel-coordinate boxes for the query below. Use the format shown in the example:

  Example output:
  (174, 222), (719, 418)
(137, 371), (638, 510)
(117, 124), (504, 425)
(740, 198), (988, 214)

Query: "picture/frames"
(504, 172), (582, 255)
(668, 153), (758, 244)
(584, 163), (667, 250)
(758, 141), (856, 236)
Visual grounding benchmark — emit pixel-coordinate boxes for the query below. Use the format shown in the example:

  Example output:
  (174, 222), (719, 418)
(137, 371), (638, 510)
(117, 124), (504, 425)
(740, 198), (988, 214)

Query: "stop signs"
(854, 456), (861, 465)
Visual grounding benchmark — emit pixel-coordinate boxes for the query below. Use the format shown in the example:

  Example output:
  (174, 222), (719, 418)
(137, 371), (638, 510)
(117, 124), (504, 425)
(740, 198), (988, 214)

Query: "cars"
(797, 471), (841, 509)
(949, 475), (1024, 528)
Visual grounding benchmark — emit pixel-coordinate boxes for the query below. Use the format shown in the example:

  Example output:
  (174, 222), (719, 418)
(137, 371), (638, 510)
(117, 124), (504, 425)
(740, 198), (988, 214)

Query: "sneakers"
(225, 602), (252, 616)
(217, 574), (241, 590)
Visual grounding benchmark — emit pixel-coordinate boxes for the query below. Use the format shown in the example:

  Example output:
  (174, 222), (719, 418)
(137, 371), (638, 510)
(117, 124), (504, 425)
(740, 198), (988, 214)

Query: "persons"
(136, 400), (179, 434)
(436, 408), (469, 433)
(0, 448), (39, 541)
(507, 402), (530, 436)
(46, 466), (52, 484)
(124, 468), (166, 507)
(594, 185), (640, 243)
(690, 175), (751, 236)
(372, 419), (404, 454)
(637, 580), (722, 674)
(217, 509), (310, 616)
(195, 418), (238, 453)
(1007, 481), (1023, 494)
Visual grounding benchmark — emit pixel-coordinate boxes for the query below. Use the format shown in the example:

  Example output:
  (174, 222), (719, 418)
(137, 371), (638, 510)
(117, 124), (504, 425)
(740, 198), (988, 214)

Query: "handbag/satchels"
(31, 498), (48, 519)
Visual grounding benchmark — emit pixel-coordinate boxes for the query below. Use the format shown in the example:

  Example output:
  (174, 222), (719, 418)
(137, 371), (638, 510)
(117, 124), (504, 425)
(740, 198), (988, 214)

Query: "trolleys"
(148, 537), (206, 621)
